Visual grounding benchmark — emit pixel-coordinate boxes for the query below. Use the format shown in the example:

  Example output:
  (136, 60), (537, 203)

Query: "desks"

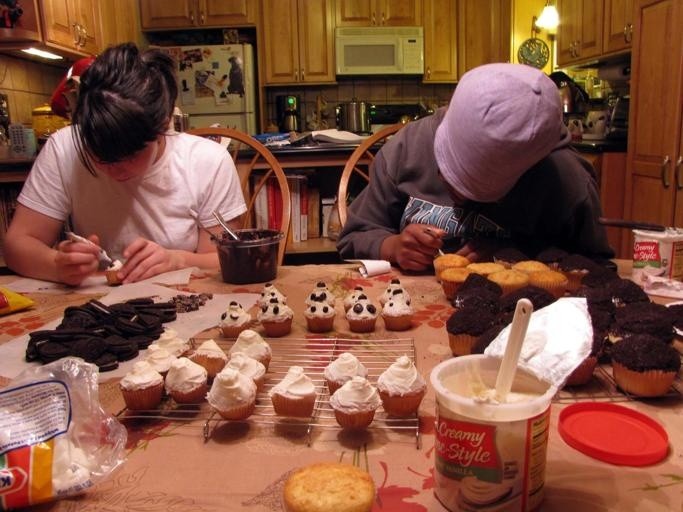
(0, 259), (682, 512)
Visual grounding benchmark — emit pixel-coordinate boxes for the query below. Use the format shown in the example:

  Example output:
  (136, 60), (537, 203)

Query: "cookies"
(25, 298), (177, 372)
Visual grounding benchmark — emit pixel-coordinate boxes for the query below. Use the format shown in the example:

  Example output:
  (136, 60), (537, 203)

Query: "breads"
(284, 463), (376, 512)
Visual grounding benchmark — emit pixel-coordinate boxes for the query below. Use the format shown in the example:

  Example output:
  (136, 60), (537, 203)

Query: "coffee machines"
(276, 94), (302, 135)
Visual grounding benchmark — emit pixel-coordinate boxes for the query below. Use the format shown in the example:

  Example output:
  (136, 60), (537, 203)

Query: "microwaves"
(334, 26), (426, 75)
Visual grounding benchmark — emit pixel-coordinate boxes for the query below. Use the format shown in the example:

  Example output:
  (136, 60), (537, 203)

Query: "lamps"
(533, 1), (561, 35)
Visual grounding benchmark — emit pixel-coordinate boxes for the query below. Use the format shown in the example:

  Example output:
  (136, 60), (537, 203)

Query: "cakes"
(105, 259), (124, 285)
(434, 246), (681, 398)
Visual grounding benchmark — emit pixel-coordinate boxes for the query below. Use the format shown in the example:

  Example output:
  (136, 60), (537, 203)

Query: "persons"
(2, 42), (248, 287)
(335, 61), (610, 274)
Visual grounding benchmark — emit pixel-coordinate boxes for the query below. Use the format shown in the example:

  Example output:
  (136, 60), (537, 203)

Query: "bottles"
(23, 128), (36, 158)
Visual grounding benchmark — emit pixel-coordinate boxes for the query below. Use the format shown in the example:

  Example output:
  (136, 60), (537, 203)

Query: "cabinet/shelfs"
(332, 0), (423, 30)
(604, 0), (632, 63)
(0, 0), (41, 50)
(139, 0), (255, 31)
(553, 0), (601, 66)
(253, 1), (336, 84)
(93, 0), (141, 54)
(459, 0), (509, 83)
(422, 0), (457, 85)
(620, 0), (682, 259)
(35, 0), (99, 61)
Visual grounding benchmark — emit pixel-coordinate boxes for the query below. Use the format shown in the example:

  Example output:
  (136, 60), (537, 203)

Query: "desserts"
(120, 279), (428, 430)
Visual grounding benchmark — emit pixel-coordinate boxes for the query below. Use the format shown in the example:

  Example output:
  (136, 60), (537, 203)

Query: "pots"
(31, 103), (66, 136)
(337, 98), (374, 135)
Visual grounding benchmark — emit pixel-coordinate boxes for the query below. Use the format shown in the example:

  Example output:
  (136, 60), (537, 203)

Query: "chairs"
(177, 126), (292, 266)
(335, 116), (416, 232)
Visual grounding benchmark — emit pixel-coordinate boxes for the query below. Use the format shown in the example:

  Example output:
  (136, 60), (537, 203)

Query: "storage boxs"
(305, 178), (335, 239)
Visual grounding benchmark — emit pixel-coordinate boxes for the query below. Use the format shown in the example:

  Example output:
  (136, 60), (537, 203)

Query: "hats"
(433, 62), (563, 203)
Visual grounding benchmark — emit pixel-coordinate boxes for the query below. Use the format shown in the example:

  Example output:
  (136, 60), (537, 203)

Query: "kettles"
(557, 80), (590, 114)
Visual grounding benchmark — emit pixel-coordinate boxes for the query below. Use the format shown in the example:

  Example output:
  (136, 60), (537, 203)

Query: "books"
(250, 167), (321, 242)
(252, 128), (364, 147)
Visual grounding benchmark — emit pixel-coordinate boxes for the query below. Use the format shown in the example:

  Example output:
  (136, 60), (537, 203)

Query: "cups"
(631, 229), (683, 281)
(210, 228), (283, 285)
(430, 353), (549, 512)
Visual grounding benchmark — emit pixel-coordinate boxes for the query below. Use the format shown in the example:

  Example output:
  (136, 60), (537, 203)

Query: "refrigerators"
(149, 42), (258, 138)
